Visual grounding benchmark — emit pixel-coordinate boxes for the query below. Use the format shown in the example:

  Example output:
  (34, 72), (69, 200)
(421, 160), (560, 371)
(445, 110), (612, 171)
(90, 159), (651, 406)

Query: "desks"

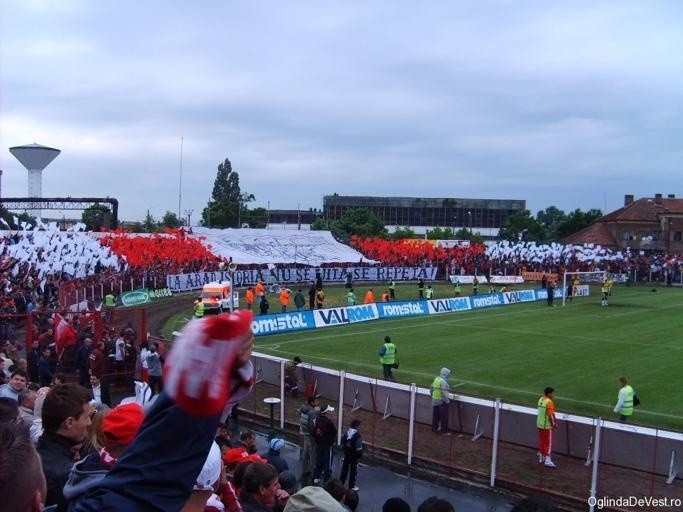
(263, 396), (281, 424)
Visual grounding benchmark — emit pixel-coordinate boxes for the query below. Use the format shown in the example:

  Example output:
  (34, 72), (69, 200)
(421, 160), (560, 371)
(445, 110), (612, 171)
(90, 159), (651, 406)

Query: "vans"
(194, 279), (239, 314)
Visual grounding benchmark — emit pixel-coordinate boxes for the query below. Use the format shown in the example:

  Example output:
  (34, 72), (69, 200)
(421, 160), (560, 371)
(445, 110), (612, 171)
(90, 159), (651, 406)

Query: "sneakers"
(352, 486), (359, 491)
(538, 454), (555, 467)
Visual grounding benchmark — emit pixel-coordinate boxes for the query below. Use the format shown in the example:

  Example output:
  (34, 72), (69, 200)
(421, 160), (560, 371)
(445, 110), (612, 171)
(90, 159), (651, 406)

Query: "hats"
(269, 438), (285, 452)
(193, 439), (268, 491)
(101, 403), (143, 446)
(321, 404), (334, 412)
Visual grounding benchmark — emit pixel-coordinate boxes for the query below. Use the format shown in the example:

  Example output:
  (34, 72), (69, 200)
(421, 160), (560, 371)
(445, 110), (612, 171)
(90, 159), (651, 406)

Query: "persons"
(352, 234), (682, 308)
(416, 495), (457, 511)
(506, 492), (563, 510)
(533, 385), (561, 469)
(377, 333), (399, 383)
(611, 374), (641, 424)
(428, 366), (457, 438)
(0, 227), (366, 511)
(381, 493), (411, 510)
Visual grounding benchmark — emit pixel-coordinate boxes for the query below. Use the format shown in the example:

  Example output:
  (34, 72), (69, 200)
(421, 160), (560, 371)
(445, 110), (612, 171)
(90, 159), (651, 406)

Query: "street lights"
(467, 211), (473, 233)
(184, 208), (195, 227)
